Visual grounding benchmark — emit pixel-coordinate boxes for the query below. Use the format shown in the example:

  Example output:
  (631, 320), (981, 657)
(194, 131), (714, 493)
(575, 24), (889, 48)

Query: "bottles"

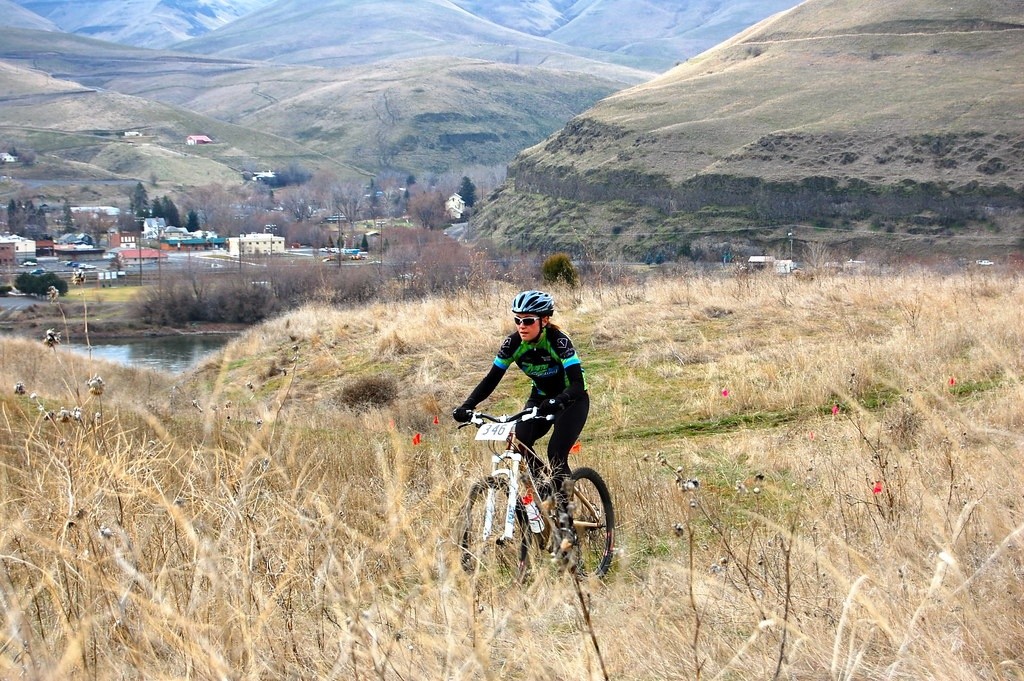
(523, 495), (545, 533)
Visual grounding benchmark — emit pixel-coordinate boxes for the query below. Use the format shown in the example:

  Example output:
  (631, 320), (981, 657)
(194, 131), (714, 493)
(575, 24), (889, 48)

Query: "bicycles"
(454, 400), (615, 592)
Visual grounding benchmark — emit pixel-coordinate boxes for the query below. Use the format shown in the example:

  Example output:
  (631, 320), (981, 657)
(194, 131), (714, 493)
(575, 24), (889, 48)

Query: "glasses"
(514, 316), (540, 325)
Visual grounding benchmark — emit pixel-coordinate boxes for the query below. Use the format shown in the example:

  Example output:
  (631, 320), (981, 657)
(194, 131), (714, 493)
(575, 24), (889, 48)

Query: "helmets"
(511, 291), (554, 317)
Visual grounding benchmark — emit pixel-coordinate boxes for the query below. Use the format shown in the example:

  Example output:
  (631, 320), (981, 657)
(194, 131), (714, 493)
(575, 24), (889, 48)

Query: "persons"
(452, 289), (588, 557)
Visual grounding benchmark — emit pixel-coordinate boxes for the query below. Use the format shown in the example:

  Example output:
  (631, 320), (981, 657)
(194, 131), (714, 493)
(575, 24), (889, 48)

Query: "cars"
(23, 260), (96, 274)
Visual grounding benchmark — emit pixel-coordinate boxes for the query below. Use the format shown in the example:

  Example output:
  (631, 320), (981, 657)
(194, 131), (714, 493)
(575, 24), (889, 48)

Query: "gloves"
(539, 399), (564, 419)
(453, 407), (472, 422)
